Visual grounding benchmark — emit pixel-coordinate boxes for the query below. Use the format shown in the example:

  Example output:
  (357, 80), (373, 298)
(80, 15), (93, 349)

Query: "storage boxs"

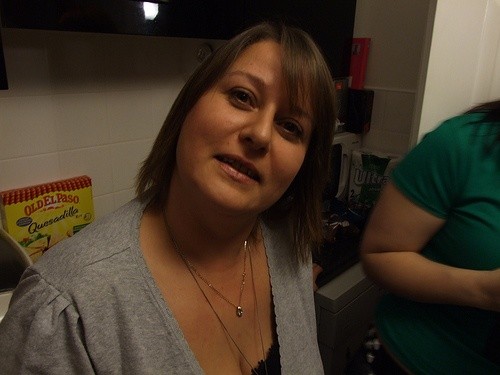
(1, 175), (95, 264)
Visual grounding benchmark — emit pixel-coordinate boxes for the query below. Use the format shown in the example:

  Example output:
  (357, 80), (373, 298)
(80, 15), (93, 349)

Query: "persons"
(350, 96), (500, 375)
(1, 18), (339, 374)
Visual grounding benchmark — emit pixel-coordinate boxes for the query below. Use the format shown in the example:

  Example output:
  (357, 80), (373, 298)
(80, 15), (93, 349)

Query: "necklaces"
(161, 211), (250, 319)
(164, 217), (269, 375)
